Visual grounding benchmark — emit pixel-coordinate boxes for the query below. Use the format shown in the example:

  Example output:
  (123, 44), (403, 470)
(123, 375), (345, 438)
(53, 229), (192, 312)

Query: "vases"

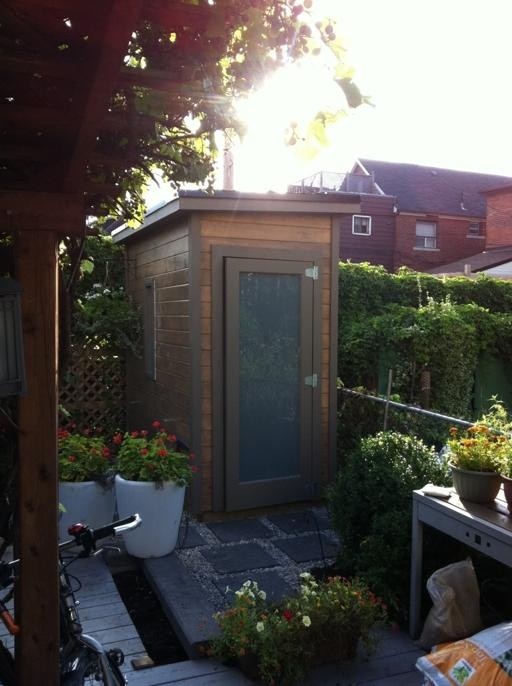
(499, 474), (511, 505)
(57, 473), (116, 553)
(308, 625), (366, 665)
(448, 459), (503, 503)
(114, 470), (187, 559)
(238, 648), (277, 682)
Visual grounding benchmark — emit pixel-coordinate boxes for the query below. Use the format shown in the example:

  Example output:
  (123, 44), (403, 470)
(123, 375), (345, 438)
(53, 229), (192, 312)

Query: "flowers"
(57, 420), (112, 482)
(487, 419), (512, 479)
(447, 392), (508, 472)
(209, 580), (313, 685)
(283, 570), (401, 656)
(112, 420), (200, 488)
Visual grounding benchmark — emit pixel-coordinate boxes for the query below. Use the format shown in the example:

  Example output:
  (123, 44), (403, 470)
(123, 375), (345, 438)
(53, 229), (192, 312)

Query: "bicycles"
(0, 513), (143, 686)
